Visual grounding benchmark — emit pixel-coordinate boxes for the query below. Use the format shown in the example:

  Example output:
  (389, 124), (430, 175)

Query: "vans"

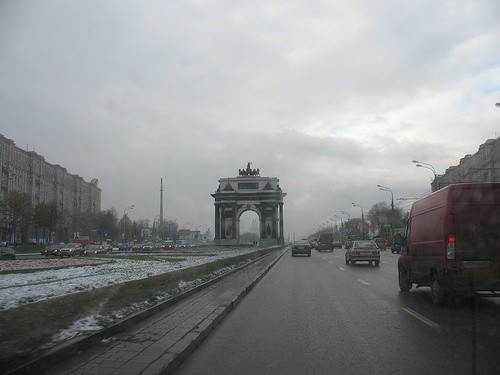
(373, 238), (388, 252)
(316, 232), (334, 252)
(391, 181), (500, 301)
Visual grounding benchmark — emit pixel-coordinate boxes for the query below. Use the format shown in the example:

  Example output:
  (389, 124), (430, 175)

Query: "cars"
(0, 246), (16, 260)
(333, 241), (343, 249)
(291, 237), (316, 257)
(39, 239), (196, 259)
(344, 240), (381, 266)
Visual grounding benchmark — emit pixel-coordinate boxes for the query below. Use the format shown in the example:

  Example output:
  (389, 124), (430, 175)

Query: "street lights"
(377, 184), (394, 234)
(341, 210), (352, 239)
(412, 159), (437, 192)
(351, 201), (365, 241)
